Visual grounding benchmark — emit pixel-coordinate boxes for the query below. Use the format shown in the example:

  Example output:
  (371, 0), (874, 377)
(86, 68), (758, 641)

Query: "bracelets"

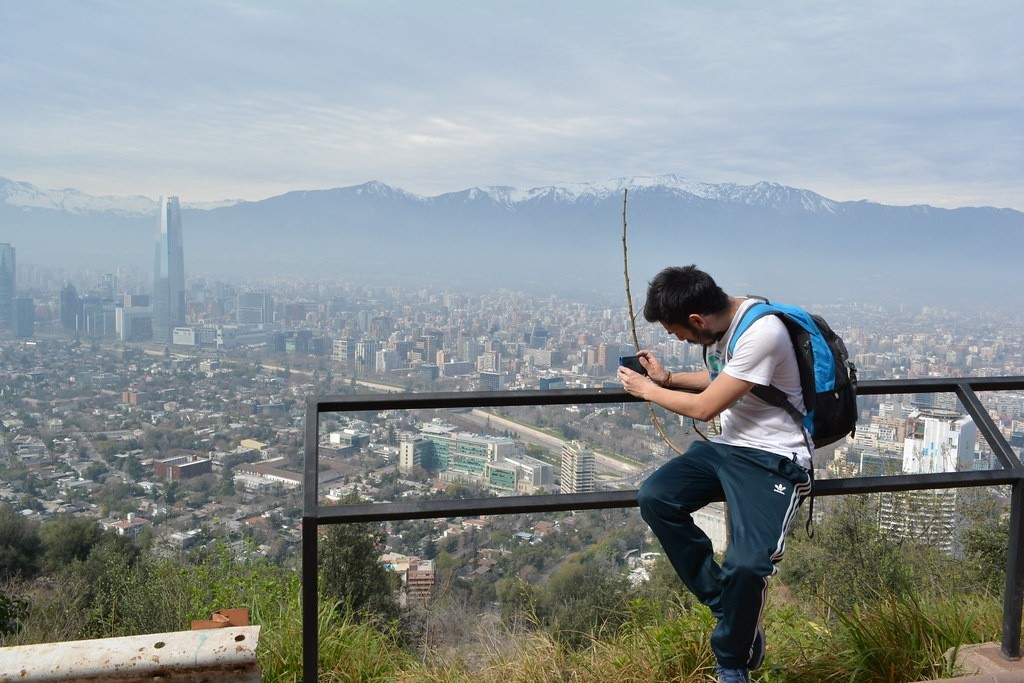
(661, 370), (672, 386)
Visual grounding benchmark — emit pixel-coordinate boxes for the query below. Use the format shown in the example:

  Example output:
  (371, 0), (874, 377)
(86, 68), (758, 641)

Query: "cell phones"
(619, 355), (649, 374)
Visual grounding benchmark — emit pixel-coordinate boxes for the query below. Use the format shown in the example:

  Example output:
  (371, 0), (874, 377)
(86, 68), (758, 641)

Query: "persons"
(617, 264), (815, 683)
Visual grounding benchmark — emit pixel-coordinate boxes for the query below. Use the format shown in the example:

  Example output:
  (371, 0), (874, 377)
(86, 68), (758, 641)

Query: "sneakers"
(716, 622), (766, 683)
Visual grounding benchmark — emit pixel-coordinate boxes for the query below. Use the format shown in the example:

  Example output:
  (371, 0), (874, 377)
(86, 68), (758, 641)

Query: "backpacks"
(727, 294), (859, 450)
